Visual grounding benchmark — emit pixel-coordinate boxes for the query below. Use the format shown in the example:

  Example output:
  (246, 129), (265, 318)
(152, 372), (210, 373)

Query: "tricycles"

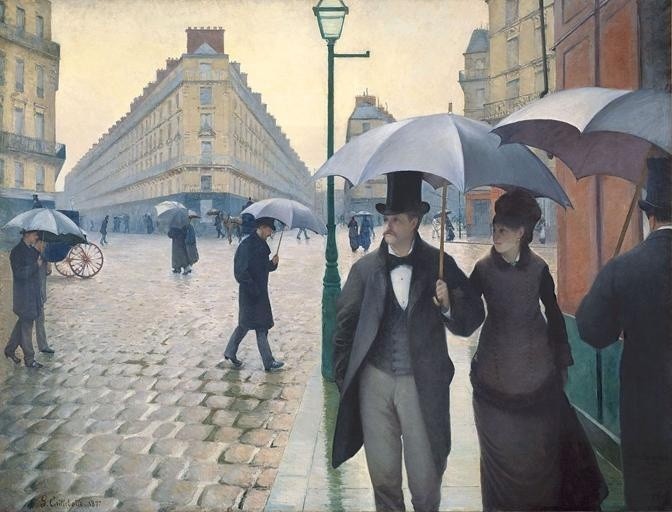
(42, 208), (105, 279)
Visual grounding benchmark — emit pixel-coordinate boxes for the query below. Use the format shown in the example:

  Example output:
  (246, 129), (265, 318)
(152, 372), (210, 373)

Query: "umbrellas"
(155, 201), (190, 229)
(39, 226), (86, 242)
(207, 208), (221, 216)
(301, 103), (570, 304)
(240, 197), (331, 257)
(434, 210), (451, 219)
(489, 83), (670, 262)
(2, 203), (87, 244)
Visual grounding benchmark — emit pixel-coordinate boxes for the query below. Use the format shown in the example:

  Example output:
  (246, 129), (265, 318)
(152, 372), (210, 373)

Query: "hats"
(376, 170), (430, 214)
(638, 157), (672, 214)
(257, 217), (275, 230)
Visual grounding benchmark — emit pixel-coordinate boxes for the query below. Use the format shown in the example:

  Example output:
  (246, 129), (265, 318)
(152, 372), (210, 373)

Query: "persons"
(30, 230), (55, 354)
(336, 210), (385, 251)
(576, 205), (672, 512)
(536, 215), (547, 243)
(144, 213), (165, 234)
(4, 225), (44, 368)
(436, 193), (608, 510)
(185, 217), (199, 273)
(330, 208), (487, 511)
(297, 226), (310, 241)
(214, 213), (225, 238)
(246, 196), (254, 207)
(225, 218), (288, 371)
(432, 217), (441, 239)
(100, 215), (110, 245)
(445, 216), (455, 241)
(112, 214), (130, 233)
(168, 226), (189, 274)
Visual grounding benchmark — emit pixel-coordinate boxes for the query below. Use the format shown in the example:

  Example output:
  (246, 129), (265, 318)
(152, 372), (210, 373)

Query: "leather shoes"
(4, 346), (54, 368)
(224, 354), (242, 366)
(265, 360), (283, 370)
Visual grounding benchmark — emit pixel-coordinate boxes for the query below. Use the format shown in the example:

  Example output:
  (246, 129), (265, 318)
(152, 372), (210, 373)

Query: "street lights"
(312, 0), (350, 386)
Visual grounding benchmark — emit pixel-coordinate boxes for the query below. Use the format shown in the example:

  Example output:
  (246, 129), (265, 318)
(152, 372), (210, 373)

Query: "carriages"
(208, 198), (277, 245)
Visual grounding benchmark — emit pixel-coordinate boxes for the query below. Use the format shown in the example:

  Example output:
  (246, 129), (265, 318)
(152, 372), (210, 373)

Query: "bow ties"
(386, 253), (418, 270)
